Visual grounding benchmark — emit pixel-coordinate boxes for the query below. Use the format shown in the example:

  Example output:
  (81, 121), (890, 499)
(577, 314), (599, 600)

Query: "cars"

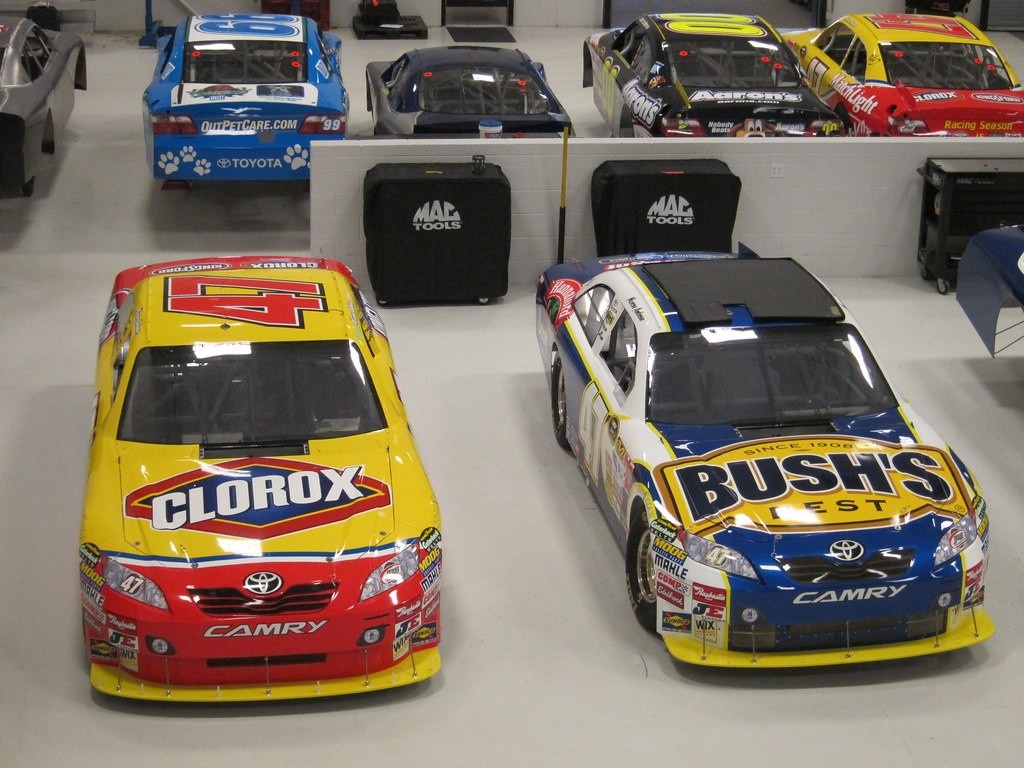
(583, 13), (848, 138)
(366, 45), (576, 139)
(536, 241), (996, 667)
(79, 255), (442, 702)
(142, 12), (350, 191)
(0, 16), (86, 198)
(781, 12), (1024, 138)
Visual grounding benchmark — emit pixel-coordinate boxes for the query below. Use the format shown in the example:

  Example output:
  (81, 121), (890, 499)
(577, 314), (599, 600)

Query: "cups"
(471, 154), (486, 173)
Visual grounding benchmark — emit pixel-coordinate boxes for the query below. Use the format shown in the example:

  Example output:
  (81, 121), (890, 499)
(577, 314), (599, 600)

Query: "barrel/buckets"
(479, 120), (503, 139)
